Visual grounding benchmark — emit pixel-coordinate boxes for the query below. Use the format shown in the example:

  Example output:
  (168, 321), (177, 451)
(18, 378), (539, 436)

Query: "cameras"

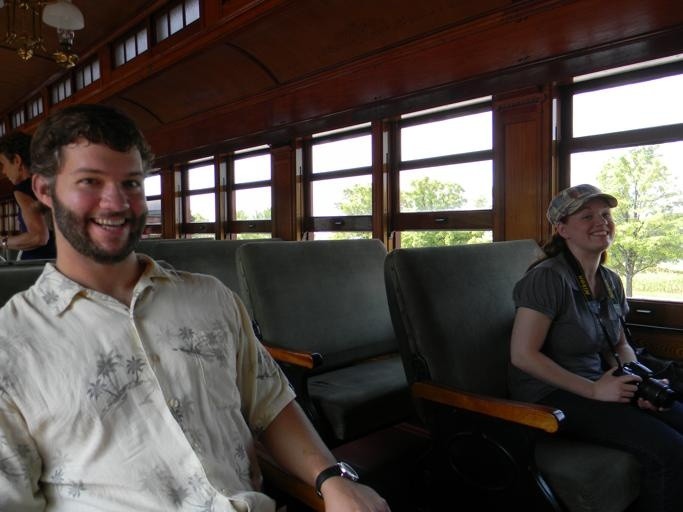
(612, 359), (675, 411)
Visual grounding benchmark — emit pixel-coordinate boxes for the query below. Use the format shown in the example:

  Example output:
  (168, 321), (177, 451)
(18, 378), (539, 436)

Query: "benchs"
(381, 238), (675, 511)
(2, 235), (283, 306)
(230, 238), (407, 447)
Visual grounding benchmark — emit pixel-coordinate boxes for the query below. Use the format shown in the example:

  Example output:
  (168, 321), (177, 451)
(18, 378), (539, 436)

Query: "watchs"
(316, 461), (360, 497)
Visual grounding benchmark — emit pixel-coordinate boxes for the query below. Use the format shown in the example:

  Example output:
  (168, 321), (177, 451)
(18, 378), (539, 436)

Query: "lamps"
(2, 0), (85, 75)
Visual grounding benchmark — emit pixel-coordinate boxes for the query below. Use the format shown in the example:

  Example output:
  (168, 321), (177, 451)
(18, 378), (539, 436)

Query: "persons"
(509, 184), (683, 512)
(0, 103), (391, 512)
(0, 131), (55, 259)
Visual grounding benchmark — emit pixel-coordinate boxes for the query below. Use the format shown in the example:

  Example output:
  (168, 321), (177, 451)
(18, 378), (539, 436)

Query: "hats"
(546, 184), (618, 227)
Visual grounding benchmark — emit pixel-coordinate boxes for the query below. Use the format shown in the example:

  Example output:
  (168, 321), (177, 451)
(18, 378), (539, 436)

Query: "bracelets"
(2, 234), (8, 250)
